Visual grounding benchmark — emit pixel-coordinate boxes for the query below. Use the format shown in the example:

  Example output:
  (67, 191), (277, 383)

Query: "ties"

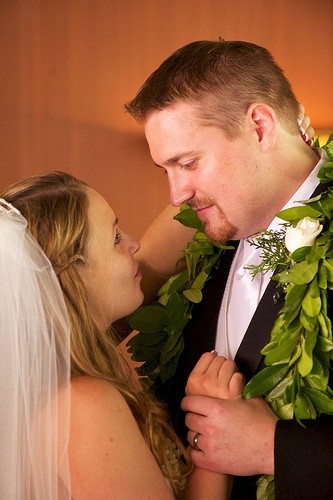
(224, 240), (270, 358)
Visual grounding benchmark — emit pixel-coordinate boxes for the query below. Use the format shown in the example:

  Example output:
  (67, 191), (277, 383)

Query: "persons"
(123, 36), (333, 500)
(0, 101), (315, 500)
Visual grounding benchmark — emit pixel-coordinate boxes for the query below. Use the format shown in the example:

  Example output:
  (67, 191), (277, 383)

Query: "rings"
(193, 432), (201, 449)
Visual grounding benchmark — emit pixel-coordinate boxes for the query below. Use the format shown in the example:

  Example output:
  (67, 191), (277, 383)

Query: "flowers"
(243, 217), (323, 282)
